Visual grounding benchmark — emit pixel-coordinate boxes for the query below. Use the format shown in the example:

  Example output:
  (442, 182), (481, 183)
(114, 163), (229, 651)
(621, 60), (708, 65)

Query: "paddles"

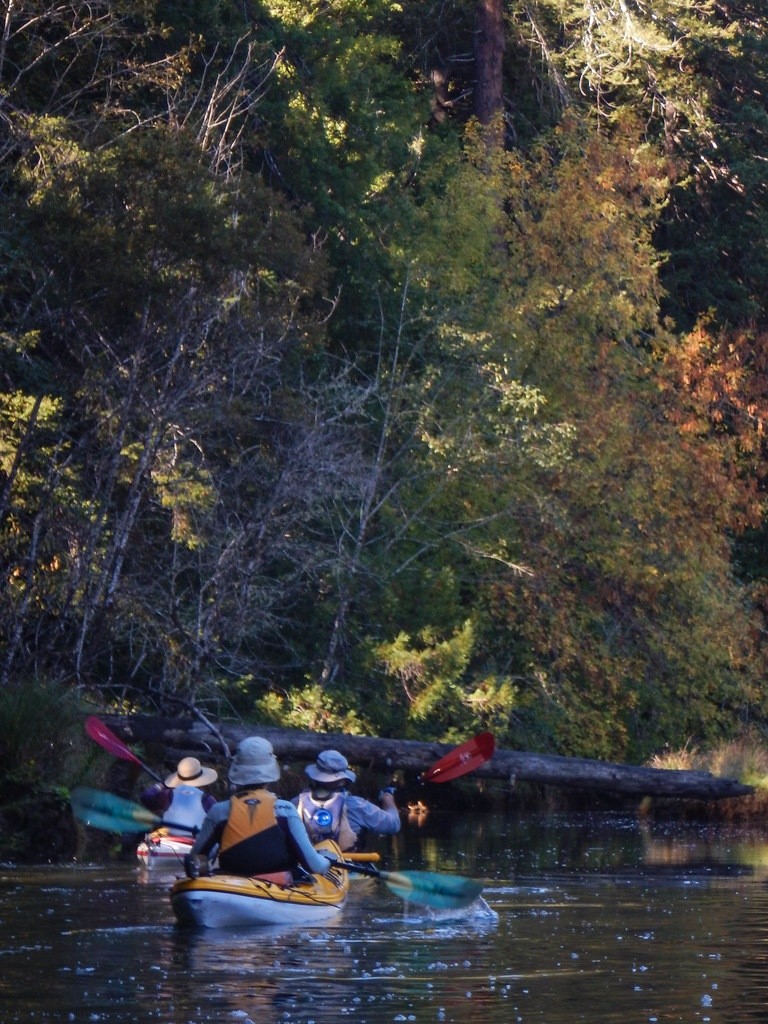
(83, 715), (164, 784)
(332, 862), (485, 909)
(69, 787), (198, 836)
(374, 731), (496, 807)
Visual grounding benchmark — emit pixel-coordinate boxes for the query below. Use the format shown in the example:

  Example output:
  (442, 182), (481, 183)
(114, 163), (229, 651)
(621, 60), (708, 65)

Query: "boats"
(136, 831), (191, 870)
(169, 837), (347, 929)
(347, 861), (378, 891)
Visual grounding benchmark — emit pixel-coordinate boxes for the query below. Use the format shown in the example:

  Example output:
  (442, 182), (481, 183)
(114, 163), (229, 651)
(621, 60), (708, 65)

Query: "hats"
(228, 737), (281, 784)
(164, 757), (218, 787)
(305, 750), (357, 783)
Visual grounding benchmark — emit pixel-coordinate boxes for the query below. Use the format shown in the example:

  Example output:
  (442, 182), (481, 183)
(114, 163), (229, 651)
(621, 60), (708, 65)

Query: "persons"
(139, 757), (219, 838)
(290, 750), (403, 853)
(190, 735), (340, 878)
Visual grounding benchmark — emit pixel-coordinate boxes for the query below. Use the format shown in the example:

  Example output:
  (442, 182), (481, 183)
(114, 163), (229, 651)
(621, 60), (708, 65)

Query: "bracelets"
(155, 785), (162, 792)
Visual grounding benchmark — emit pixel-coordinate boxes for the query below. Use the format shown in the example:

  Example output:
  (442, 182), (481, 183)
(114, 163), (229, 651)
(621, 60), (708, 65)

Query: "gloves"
(377, 786), (396, 801)
(318, 850), (338, 867)
